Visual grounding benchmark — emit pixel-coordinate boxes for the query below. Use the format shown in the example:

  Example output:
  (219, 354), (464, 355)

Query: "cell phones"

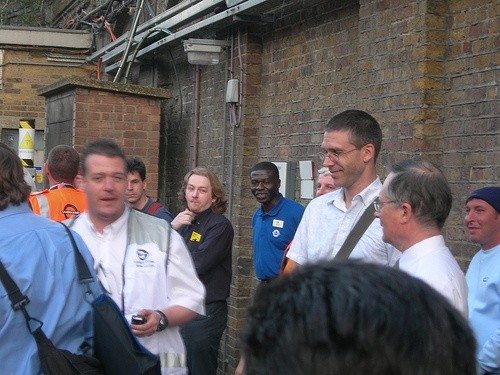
(132, 315), (145, 324)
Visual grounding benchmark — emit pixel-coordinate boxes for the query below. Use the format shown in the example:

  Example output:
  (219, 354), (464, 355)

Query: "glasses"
(322, 145), (363, 163)
(373, 200), (412, 213)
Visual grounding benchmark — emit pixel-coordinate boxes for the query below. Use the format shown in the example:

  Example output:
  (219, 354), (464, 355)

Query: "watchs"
(157, 310), (168, 331)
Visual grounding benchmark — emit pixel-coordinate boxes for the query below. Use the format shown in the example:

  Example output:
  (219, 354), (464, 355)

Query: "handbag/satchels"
(0, 221), (161, 375)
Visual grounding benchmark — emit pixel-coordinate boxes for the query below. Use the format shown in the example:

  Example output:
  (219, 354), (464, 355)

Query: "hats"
(465, 187), (500, 215)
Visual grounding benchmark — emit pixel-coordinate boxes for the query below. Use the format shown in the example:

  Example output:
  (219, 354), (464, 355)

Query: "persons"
(171, 166), (234, 375)
(464, 186), (500, 375)
(0, 142), (104, 375)
(279, 167), (342, 278)
(126, 159), (176, 223)
(373, 158), (469, 320)
(28, 145), (88, 223)
(235, 257), (479, 375)
(286, 109), (402, 266)
(249, 161), (306, 281)
(62, 140), (207, 375)
